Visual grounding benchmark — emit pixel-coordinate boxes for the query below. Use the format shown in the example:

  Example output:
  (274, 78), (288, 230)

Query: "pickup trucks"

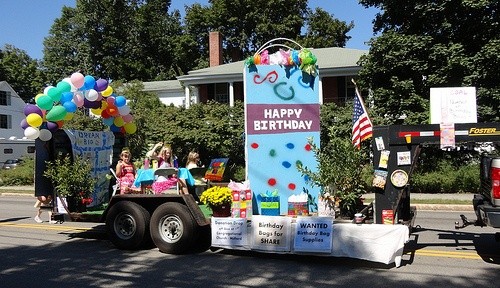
(471, 151), (500, 265)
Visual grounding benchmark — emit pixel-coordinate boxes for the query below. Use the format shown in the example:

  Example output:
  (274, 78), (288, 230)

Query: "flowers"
(79, 191), (93, 205)
(200, 184), (234, 209)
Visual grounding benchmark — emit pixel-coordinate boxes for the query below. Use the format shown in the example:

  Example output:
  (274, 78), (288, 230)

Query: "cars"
(2, 159), (26, 170)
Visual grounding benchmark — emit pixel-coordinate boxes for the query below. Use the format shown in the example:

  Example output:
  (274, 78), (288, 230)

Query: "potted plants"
(295, 120), (377, 218)
(43, 150), (97, 213)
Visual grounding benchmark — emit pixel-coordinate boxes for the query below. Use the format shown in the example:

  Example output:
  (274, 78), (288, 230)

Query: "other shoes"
(34, 216), (43, 223)
(49, 221), (58, 224)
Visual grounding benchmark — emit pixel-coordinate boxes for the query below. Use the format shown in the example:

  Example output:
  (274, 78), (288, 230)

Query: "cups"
(355, 213), (363, 224)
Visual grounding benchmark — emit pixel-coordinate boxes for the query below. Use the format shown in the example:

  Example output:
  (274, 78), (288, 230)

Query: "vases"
(210, 204), (232, 218)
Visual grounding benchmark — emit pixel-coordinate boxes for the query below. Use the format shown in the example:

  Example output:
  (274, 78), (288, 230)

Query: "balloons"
(21, 72), (136, 141)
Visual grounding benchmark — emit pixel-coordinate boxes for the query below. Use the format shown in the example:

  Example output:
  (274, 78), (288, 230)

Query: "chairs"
(153, 167), (179, 195)
(109, 168), (121, 196)
(187, 168), (210, 205)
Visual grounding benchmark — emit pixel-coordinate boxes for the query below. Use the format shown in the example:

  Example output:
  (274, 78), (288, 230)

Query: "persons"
(116, 147), (136, 188)
(34, 196), (57, 223)
(145, 142), (178, 169)
(186, 151), (200, 170)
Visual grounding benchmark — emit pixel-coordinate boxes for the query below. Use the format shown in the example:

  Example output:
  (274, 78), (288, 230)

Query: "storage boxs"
(287, 201), (310, 217)
(205, 157), (237, 181)
(260, 196), (281, 216)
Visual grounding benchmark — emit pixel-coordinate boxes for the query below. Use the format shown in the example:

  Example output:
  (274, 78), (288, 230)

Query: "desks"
(135, 167), (195, 195)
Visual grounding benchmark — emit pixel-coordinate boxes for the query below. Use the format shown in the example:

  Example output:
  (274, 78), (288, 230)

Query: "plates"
(390, 169), (408, 187)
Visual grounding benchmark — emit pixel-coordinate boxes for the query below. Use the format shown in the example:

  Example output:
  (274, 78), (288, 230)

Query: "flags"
(351, 91), (373, 150)
